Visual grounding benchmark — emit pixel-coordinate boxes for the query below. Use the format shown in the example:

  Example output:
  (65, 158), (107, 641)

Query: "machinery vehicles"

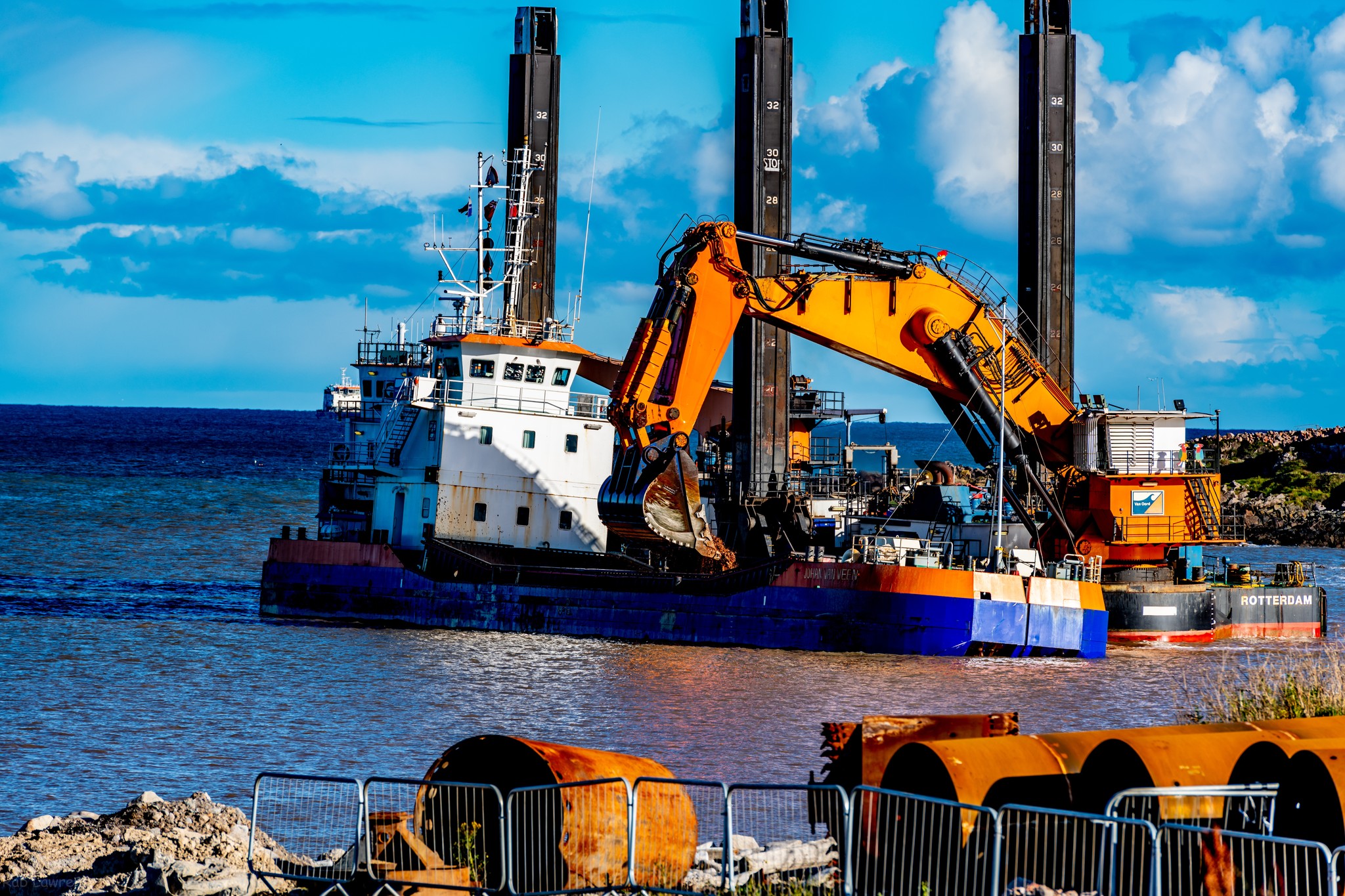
(601, 205), (1330, 652)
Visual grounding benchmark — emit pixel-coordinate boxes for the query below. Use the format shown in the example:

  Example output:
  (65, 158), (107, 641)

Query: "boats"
(258, 132), (1111, 661)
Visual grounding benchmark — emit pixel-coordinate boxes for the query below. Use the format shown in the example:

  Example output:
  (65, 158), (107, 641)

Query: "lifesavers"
(333, 444), (350, 461)
(384, 382), (395, 400)
